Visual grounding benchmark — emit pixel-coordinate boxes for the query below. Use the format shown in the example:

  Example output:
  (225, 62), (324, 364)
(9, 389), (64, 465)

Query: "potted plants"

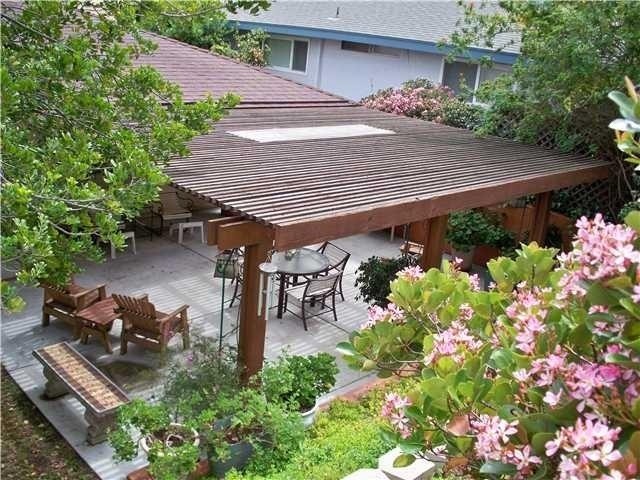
(444, 207), (520, 272)
(107, 344), (338, 479)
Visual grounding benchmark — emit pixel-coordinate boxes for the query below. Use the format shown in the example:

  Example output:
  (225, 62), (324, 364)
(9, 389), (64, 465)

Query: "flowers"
(335, 212), (640, 480)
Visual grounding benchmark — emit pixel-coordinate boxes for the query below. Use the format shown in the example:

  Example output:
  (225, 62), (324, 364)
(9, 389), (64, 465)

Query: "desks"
(73, 296), (135, 355)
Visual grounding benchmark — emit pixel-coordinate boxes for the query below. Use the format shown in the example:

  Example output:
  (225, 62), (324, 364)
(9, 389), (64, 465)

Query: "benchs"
(32, 338), (132, 448)
(398, 238), (425, 261)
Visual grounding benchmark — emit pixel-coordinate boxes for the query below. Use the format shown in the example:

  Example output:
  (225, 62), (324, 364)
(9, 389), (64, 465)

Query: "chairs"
(80, 190), (206, 261)
(228, 241), (351, 332)
(31, 270), (106, 336)
(111, 287), (194, 363)
